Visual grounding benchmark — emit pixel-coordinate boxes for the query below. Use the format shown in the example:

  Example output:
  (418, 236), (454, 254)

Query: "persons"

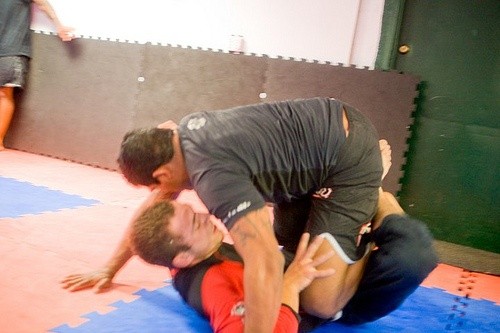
(59, 95), (394, 333)
(124, 136), (438, 333)
(0, 0), (76, 153)
